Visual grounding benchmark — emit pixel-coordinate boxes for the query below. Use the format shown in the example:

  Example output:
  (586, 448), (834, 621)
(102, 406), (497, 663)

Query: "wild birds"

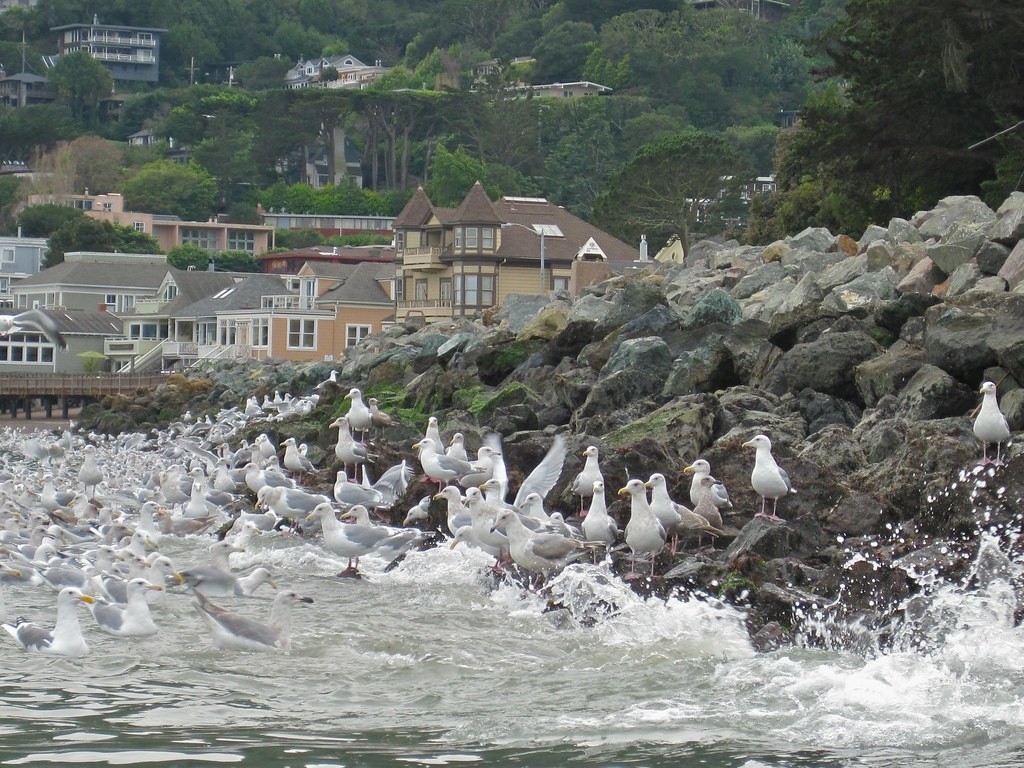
(742, 434), (797, 523)
(1, 368), (731, 659)
(973, 381), (1010, 467)
(0, 308), (69, 353)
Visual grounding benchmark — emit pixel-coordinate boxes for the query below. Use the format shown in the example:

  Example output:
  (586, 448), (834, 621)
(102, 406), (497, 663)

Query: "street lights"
(501, 222), (545, 296)
